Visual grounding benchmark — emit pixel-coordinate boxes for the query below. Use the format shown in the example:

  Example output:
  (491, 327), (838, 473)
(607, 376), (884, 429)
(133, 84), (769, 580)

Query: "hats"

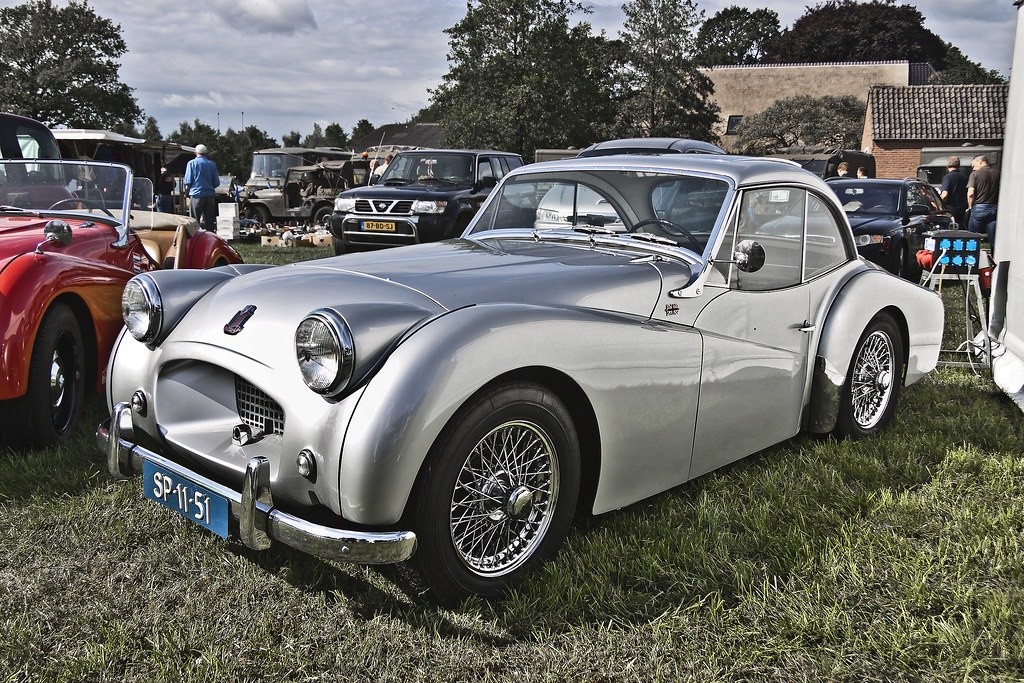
(195, 144), (208, 154)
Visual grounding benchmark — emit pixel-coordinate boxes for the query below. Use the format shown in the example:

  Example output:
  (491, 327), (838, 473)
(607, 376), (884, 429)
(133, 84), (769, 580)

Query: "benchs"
(60, 208), (197, 269)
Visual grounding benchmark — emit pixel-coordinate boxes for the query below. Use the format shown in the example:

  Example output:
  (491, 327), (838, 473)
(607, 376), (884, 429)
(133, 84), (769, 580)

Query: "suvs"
(752, 176), (957, 285)
(328, 145), (536, 257)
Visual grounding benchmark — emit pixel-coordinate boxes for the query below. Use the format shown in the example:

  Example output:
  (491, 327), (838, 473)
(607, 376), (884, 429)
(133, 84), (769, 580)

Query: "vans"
(534, 135), (736, 239)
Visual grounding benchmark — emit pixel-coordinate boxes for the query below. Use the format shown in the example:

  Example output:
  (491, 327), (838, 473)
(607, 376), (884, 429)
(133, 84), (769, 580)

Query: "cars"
(93, 146), (950, 609)
(1, 145), (427, 451)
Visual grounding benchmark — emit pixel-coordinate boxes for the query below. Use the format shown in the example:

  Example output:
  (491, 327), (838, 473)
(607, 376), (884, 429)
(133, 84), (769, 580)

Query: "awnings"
(918, 157), (975, 173)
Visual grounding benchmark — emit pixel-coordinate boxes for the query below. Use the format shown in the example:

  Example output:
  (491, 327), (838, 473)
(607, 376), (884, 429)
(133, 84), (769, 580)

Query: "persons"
(967, 154), (1000, 256)
(370, 154), (393, 176)
(940, 157), (968, 230)
(183, 144), (220, 233)
(837, 162), (868, 179)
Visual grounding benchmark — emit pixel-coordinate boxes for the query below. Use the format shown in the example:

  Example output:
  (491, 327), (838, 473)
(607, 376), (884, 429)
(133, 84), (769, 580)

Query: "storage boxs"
(261, 233), (336, 248)
